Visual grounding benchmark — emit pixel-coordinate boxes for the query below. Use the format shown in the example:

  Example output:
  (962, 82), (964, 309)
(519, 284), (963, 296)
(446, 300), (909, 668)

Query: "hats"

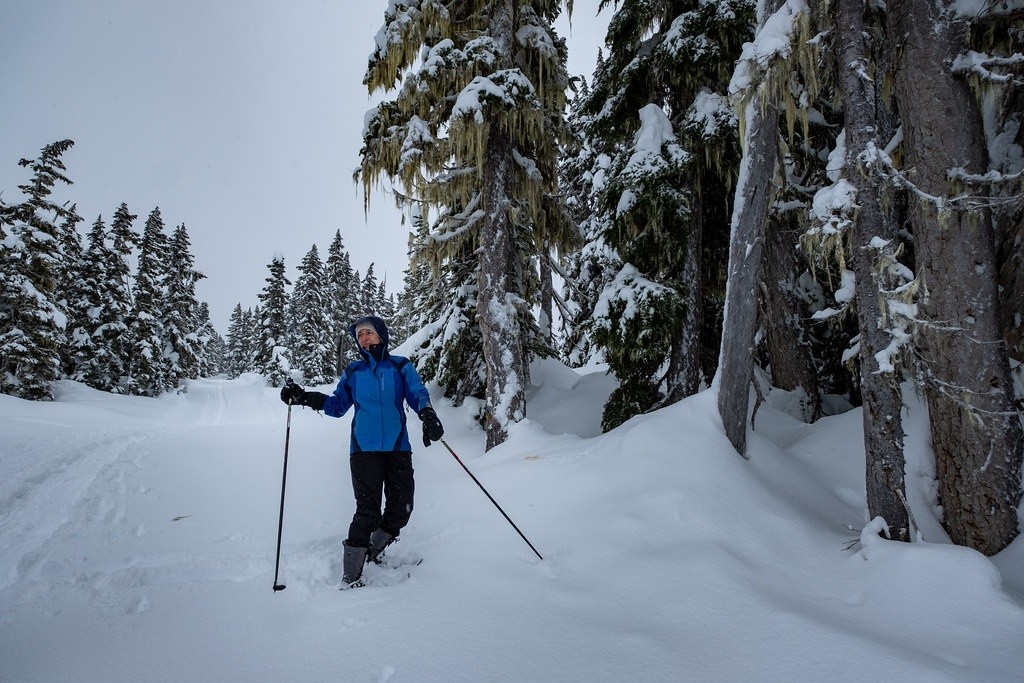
(355, 321), (382, 341)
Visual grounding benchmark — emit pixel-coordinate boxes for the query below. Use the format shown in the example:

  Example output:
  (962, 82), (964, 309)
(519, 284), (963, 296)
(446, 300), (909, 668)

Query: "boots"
(366, 527), (400, 564)
(340, 539), (365, 590)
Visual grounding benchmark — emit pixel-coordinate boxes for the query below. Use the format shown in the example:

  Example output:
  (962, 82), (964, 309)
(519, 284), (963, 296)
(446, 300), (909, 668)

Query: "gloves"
(418, 407), (444, 447)
(281, 377), (324, 417)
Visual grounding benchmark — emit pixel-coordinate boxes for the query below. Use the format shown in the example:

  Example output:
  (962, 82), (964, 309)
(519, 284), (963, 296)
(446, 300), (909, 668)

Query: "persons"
(280, 316), (445, 590)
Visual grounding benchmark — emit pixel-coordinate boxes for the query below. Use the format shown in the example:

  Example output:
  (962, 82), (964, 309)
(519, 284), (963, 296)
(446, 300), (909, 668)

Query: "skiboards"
(332, 551), (423, 592)
